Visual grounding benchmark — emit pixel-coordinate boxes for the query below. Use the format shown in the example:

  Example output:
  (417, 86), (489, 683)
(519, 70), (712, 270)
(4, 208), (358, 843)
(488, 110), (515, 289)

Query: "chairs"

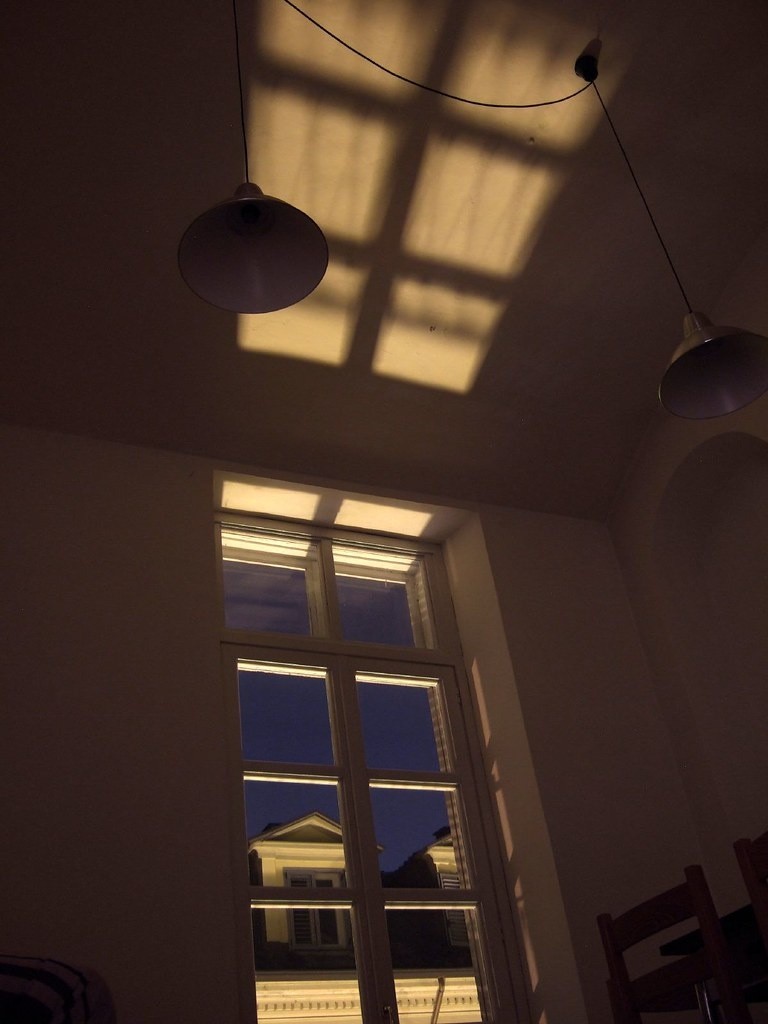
(733, 828), (768, 947)
(596, 865), (768, 1024)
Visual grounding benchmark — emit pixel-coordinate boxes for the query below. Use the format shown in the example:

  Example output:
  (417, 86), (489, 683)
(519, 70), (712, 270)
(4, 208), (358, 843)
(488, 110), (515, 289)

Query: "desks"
(658, 903), (768, 1002)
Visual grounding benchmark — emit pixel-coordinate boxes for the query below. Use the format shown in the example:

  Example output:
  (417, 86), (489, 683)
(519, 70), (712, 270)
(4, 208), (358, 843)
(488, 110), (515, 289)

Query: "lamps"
(176, 0), (329, 317)
(576, 54), (768, 421)
